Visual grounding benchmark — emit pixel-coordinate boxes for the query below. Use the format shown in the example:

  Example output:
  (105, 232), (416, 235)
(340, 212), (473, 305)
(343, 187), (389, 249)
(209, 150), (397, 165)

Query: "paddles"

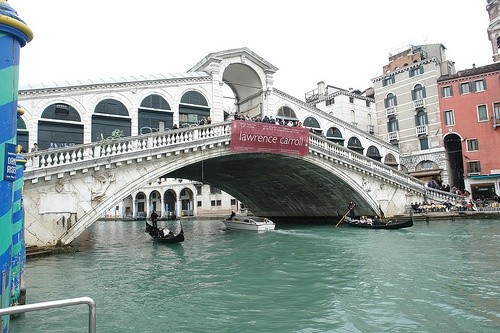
(134, 220), (155, 240)
(335, 204), (355, 227)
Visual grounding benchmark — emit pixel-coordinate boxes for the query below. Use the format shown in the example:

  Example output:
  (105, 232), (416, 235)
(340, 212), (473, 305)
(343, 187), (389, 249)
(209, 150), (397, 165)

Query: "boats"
(221, 216), (276, 231)
(145, 221), (184, 244)
(336, 210), (414, 230)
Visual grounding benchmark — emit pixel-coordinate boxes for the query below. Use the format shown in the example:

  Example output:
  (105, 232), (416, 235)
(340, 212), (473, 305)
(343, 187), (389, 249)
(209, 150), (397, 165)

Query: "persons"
(360, 215), (380, 225)
(229, 211), (235, 217)
(428, 182), (470, 200)
(49, 142), (75, 148)
(474, 193), (500, 203)
(161, 226), (170, 238)
(199, 116), (211, 138)
(276, 119), (302, 126)
(348, 201), (356, 220)
(171, 124), (179, 143)
(411, 200), (452, 213)
(455, 199), (489, 211)
(17, 143), (41, 153)
(234, 111), (275, 124)
(310, 128), (313, 140)
(151, 210), (158, 228)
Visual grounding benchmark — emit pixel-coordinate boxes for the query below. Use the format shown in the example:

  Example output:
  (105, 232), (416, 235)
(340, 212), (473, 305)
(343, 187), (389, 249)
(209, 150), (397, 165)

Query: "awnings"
(408, 170), (442, 177)
(471, 175), (500, 180)
